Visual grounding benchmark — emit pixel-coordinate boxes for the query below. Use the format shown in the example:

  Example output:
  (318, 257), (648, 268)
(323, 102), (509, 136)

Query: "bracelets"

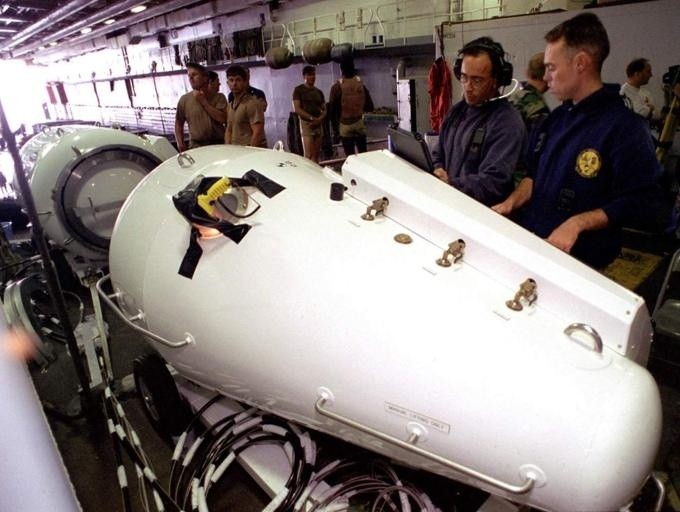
(308, 115), (313, 122)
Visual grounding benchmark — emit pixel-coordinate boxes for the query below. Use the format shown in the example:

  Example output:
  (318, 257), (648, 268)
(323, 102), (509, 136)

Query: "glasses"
(457, 73), (487, 89)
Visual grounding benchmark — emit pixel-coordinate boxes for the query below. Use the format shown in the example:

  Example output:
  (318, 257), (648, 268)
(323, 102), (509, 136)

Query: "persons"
(173, 62), (227, 154)
(431, 35), (528, 220)
(489, 11), (664, 273)
(617, 58), (657, 125)
(206, 69), (221, 93)
(328, 56), (375, 158)
(292, 65), (328, 164)
(227, 62), (268, 113)
(507, 50), (551, 219)
(222, 65), (267, 148)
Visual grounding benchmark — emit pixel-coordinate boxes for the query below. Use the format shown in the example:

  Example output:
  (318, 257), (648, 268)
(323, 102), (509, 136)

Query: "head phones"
(452, 41), (514, 88)
